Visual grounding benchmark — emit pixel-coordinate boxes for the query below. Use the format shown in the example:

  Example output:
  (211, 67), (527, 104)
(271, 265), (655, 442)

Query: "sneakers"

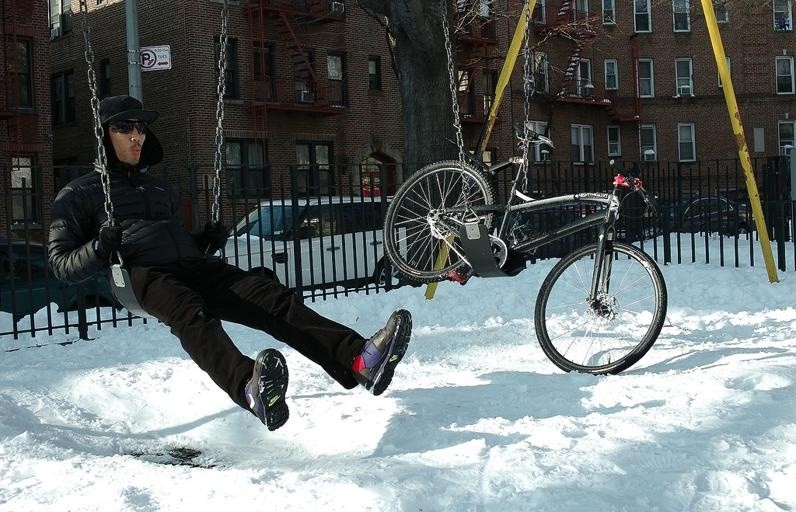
(348, 307), (412, 396)
(243, 347), (290, 432)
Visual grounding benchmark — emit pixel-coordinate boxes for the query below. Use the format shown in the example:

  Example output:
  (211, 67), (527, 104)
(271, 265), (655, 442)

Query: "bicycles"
(382, 120), (669, 377)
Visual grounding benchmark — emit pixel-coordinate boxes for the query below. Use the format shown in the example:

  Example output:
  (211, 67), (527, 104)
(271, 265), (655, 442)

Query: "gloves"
(96, 221), (125, 259)
(200, 220), (230, 251)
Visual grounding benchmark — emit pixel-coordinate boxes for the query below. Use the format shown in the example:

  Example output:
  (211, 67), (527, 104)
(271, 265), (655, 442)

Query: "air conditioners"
(50, 27), (61, 38)
(328, 1), (345, 16)
(644, 150), (657, 161)
(297, 90), (316, 103)
(681, 85), (693, 95)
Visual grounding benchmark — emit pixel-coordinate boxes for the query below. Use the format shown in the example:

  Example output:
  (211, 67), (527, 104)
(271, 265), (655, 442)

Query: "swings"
(442, 0), (531, 277)
(80, 1), (231, 315)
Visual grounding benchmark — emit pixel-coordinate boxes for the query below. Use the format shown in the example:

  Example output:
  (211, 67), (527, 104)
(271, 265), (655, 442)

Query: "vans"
(212, 194), (409, 288)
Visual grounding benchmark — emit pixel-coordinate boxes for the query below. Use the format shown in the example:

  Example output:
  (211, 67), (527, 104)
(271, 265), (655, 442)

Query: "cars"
(658, 194), (753, 236)
(492, 208), (596, 263)
(0, 239), (126, 324)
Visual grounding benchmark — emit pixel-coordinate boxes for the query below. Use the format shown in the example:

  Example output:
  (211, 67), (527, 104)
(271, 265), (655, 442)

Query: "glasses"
(103, 117), (152, 134)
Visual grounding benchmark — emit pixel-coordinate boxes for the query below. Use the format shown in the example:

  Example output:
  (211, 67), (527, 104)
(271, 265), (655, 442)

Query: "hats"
(90, 96), (160, 132)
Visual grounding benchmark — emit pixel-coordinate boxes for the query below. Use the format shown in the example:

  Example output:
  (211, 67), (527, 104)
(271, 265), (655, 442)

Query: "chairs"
(311, 211), (336, 236)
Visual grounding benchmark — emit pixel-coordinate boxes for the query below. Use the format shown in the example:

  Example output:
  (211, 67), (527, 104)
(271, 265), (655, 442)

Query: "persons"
(47, 94), (412, 432)
